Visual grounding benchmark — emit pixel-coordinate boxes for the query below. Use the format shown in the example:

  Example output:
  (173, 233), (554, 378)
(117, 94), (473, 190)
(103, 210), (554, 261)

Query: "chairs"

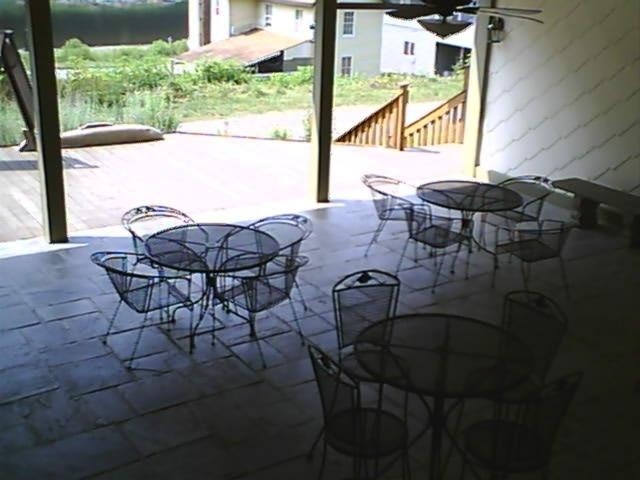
(438, 369), (585, 479)
(441, 291), (568, 458)
(494, 218), (572, 293)
(324, 268), (413, 412)
(209, 254), (310, 367)
(119, 204), (211, 320)
(359, 171), (434, 263)
(88, 250), (197, 367)
(395, 204), (473, 297)
(206, 214), (315, 339)
(477, 175), (551, 265)
(303, 346), (412, 479)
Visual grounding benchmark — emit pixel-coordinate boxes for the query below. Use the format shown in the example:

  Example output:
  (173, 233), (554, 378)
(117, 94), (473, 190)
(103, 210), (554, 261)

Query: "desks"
(142, 223), (281, 336)
(415, 179), (523, 276)
(351, 312), (538, 480)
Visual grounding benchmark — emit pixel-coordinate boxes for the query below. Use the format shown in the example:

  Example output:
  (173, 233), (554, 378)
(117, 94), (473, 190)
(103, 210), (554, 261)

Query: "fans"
(334, 0), (546, 25)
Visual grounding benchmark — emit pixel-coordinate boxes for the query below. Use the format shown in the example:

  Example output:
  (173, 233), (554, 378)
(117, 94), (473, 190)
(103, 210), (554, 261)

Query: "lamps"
(485, 17), (505, 45)
(414, 15), (473, 39)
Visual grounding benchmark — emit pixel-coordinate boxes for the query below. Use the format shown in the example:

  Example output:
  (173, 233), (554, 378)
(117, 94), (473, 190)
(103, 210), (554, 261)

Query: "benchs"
(552, 177), (640, 249)
